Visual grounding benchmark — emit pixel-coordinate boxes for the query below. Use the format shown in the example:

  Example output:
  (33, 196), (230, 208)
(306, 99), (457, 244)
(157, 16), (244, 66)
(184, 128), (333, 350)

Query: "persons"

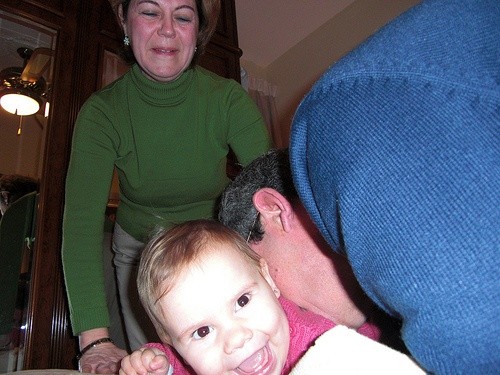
(217, 0), (500, 375)
(62, 0), (274, 375)
(119, 218), (426, 375)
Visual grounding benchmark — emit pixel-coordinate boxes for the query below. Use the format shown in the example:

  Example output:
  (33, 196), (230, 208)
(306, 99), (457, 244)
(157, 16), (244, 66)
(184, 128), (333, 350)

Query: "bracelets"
(74, 338), (114, 361)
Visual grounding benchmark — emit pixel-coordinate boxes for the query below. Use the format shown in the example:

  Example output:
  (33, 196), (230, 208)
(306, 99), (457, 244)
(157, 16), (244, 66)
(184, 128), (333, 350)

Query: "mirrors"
(0, 14), (58, 375)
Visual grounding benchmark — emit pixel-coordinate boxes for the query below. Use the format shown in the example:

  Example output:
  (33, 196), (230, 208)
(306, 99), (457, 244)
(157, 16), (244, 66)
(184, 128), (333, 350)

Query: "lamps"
(0, 47), (46, 116)
